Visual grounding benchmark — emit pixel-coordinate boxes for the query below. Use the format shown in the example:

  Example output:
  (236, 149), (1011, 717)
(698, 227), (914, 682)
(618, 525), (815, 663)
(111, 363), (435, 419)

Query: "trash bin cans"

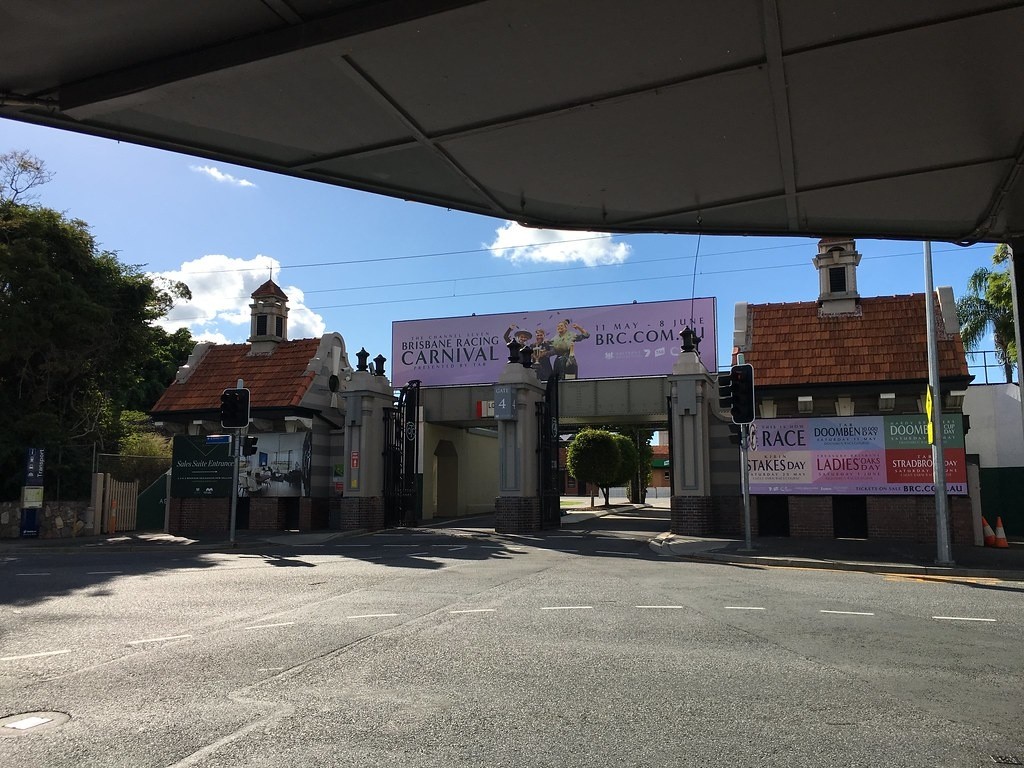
(20, 508), (40, 538)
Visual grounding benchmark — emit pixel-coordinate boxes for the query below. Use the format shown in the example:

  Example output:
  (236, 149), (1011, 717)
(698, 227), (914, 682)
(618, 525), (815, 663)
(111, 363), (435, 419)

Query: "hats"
(514, 328), (532, 340)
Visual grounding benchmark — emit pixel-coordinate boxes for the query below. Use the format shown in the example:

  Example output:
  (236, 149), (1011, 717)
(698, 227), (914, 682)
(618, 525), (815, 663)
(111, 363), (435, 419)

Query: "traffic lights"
(241, 435), (259, 458)
(730, 363), (757, 425)
(728, 424), (742, 447)
(221, 388), (251, 429)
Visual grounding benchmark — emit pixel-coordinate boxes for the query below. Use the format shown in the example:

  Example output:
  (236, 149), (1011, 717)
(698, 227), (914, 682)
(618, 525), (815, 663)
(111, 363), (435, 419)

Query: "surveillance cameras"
(340, 367), (350, 372)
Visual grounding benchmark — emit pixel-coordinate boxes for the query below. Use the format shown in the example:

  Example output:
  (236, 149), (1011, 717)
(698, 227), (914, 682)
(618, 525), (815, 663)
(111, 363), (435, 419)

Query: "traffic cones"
(982, 516), (996, 547)
(991, 516), (1010, 550)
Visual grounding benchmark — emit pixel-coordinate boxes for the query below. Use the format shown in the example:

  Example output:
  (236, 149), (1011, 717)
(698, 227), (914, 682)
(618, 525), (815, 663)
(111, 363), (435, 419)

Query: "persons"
(529, 329), (555, 380)
(504, 322), (533, 368)
(549, 319), (589, 379)
